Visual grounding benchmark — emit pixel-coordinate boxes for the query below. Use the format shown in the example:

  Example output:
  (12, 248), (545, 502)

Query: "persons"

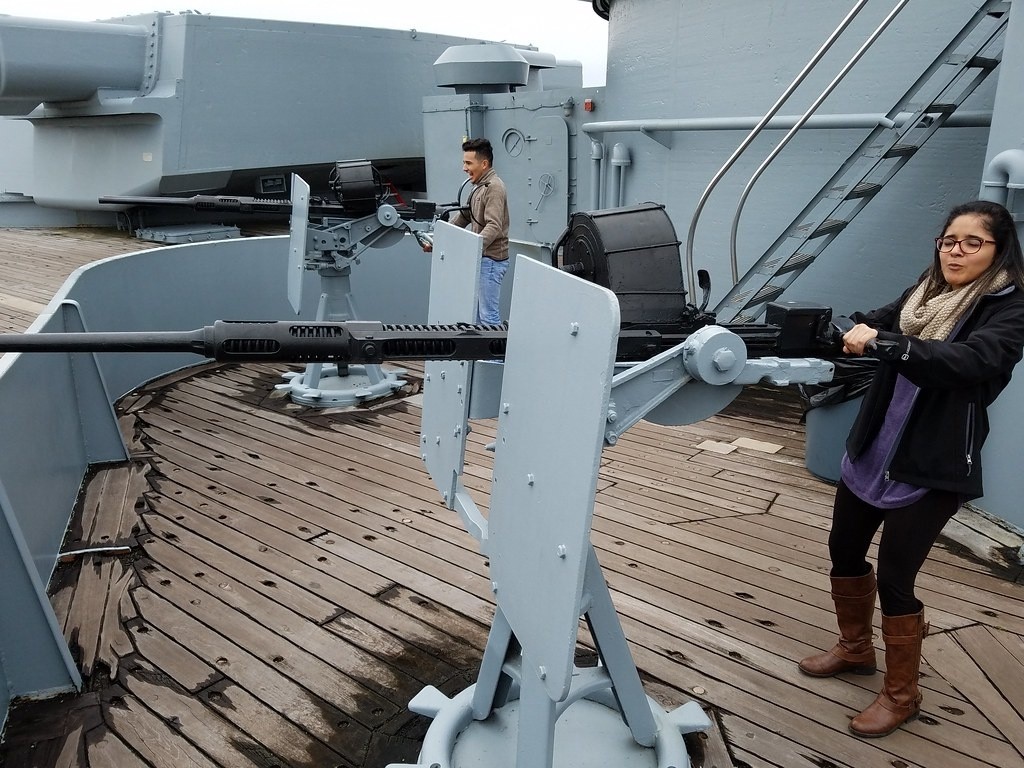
(423, 138), (510, 325)
(798, 200), (1024, 739)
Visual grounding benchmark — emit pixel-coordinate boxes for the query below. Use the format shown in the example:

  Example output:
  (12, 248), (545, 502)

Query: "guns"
(98, 181), (469, 226)
(0, 314), (899, 426)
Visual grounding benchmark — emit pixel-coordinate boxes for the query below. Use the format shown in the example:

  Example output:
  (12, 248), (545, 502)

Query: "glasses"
(935, 236), (996, 254)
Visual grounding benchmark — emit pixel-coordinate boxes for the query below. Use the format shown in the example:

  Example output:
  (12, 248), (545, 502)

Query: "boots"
(798, 562), (877, 678)
(848, 605), (930, 739)
